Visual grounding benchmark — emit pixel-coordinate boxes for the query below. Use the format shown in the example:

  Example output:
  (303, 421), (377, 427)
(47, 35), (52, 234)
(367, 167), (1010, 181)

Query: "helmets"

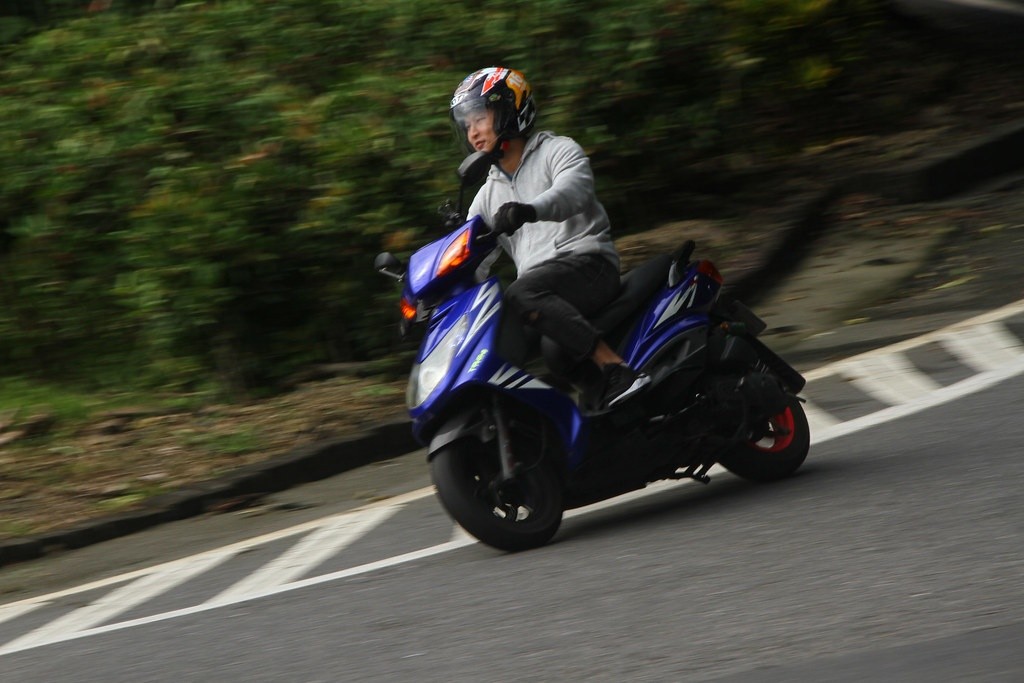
(448, 66), (537, 142)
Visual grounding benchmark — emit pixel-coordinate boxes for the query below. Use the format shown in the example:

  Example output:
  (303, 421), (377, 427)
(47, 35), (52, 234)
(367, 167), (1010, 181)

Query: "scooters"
(367, 151), (811, 554)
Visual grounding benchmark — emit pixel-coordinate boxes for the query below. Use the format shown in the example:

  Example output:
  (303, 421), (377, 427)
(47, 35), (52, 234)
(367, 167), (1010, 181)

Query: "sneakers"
(603, 355), (652, 409)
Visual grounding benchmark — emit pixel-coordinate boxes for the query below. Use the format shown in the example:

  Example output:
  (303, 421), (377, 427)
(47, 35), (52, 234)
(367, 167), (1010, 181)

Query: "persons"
(450, 67), (652, 408)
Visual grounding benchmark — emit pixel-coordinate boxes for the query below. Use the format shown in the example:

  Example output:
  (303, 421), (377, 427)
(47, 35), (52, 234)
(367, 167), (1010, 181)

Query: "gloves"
(493, 202), (537, 237)
